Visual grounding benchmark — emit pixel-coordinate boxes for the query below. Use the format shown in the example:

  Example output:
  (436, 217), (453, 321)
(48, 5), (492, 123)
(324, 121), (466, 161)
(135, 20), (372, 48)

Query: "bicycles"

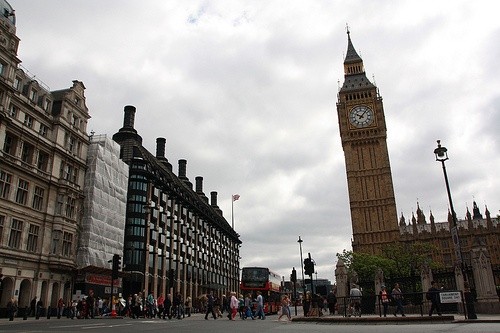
(345, 300), (361, 318)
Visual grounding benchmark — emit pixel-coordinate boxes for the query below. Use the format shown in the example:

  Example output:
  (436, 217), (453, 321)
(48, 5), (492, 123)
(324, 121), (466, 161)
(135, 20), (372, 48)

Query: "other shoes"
(402, 315), (406, 316)
(393, 313), (397, 317)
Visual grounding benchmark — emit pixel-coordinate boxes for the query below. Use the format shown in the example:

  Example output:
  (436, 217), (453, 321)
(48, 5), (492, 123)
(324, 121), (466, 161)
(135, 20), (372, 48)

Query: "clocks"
(348, 104), (374, 129)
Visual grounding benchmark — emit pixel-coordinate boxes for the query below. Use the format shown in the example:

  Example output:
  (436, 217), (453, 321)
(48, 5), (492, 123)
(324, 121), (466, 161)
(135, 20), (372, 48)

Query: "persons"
(327, 290), (337, 315)
(71, 288), (324, 320)
(428, 280), (444, 316)
(347, 283), (362, 317)
(28, 296), (43, 317)
(6, 299), (17, 321)
(57, 297), (65, 316)
(391, 283), (406, 317)
(379, 285), (391, 317)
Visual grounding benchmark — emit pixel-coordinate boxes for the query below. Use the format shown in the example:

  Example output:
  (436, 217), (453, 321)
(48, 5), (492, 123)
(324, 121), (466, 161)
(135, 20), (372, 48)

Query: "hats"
(381, 285), (386, 288)
(352, 284), (357, 287)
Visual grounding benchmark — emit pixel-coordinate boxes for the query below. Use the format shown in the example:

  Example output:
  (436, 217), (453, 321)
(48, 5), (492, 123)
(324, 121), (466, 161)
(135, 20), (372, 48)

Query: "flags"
(233, 194), (240, 201)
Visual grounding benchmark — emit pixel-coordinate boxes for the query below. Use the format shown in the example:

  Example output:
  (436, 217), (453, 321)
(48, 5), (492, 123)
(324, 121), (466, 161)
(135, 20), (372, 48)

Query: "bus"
(239, 266), (281, 314)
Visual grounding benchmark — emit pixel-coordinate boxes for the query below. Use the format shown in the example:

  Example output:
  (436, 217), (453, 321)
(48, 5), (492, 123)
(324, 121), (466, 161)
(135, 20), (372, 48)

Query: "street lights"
(434, 140), (477, 320)
(297, 236), (308, 316)
(315, 272), (317, 287)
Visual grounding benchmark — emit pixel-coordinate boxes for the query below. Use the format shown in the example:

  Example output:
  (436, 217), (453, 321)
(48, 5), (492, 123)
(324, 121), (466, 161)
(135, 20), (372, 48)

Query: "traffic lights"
(291, 270), (296, 282)
(303, 258), (310, 275)
(113, 254), (121, 279)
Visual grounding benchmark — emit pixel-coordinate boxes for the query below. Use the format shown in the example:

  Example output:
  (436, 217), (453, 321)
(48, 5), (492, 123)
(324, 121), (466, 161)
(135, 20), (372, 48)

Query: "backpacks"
(426, 292), (431, 300)
(389, 292), (398, 302)
